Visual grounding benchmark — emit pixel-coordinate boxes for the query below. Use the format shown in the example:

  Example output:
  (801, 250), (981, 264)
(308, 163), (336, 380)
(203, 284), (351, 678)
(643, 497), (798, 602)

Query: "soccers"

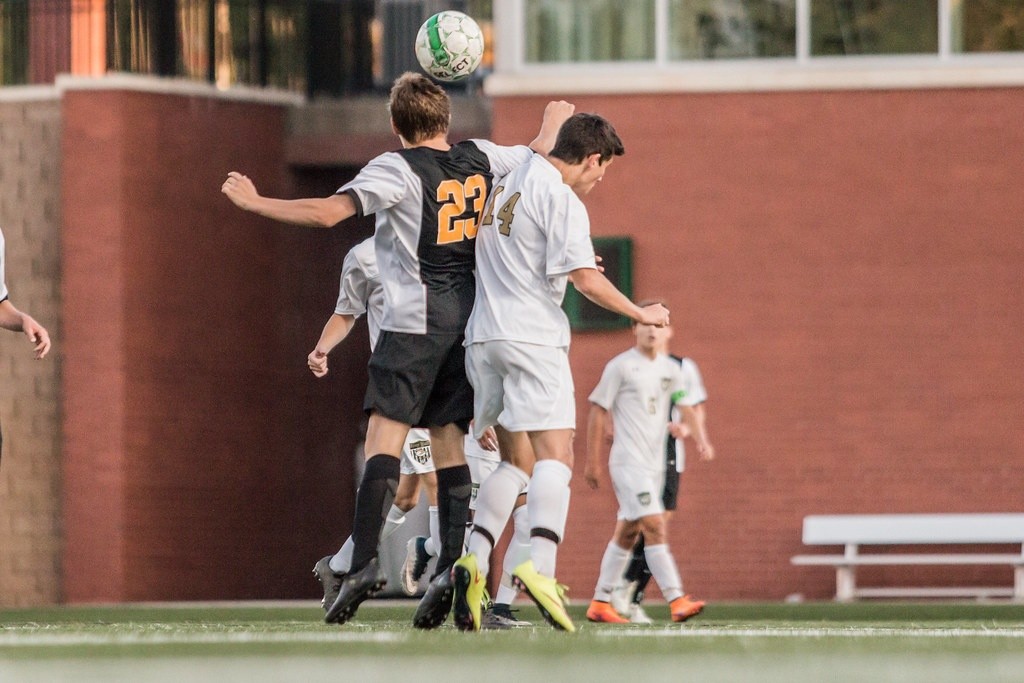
(413, 8), (489, 82)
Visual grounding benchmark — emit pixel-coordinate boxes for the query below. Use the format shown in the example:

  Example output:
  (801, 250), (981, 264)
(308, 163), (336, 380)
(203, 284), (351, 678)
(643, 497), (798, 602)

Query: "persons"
(0, 227), (51, 360)
(585, 301), (714, 625)
(454, 113), (670, 632)
(220, 73), (576, 629)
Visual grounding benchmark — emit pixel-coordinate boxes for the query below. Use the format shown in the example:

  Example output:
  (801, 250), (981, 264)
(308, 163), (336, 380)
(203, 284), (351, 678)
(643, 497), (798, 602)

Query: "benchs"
(792, 512), (1024, 603)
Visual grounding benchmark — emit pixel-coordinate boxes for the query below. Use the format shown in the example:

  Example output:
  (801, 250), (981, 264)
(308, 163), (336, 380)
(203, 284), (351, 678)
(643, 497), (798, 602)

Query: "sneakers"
(611, 580), (653, 626)
(511, 559), (576, 633)
(399, 536), (428, 596)
(322, 552), (388, 627)
(670, 593), (706, 623)
(481, 601), (533, 629)
(411, 565), (455, 631)
(585, 599), (630, 624)
(312, 555), (344, 611)
(451, 552), (486, 633)
